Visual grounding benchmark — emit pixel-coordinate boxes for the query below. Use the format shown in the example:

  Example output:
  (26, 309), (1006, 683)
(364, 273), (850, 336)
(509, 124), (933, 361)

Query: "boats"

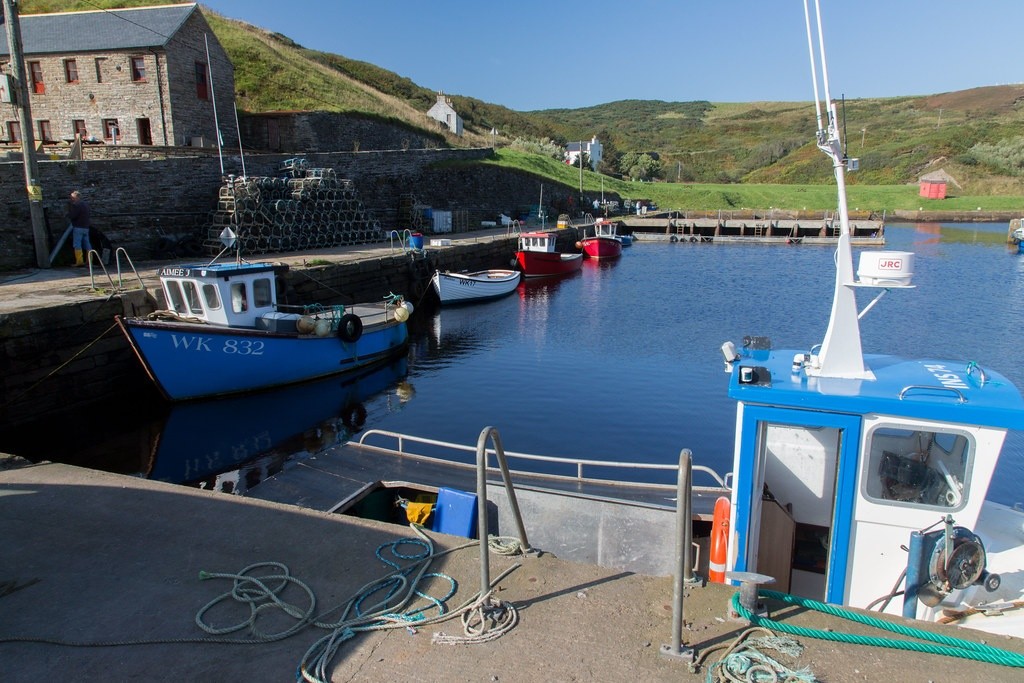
(593, 220), (632, 247)
(241, 0), (1024, 639)
(582, 220), (622, 260)
(116, 262), (417, 400)
(514, 232), (583, 277)
(431, 269), (520, 303)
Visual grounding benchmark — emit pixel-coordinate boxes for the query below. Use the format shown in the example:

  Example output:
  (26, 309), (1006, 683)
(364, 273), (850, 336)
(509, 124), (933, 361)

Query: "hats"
(71, 191), (81, 198)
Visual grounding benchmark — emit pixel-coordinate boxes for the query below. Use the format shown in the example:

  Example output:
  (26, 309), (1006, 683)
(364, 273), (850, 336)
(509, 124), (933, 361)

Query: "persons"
(65, 190), (95, 268)
(591, 199), (600, 218)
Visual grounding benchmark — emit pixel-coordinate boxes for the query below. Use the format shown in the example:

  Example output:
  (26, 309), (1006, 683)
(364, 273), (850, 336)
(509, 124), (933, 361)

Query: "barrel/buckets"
(410, 233), (423, 250)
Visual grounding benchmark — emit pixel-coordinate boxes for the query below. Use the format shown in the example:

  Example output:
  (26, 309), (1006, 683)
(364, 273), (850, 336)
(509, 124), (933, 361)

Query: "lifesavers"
(707, 496), (730, 584)
(337, 314), (363, 343)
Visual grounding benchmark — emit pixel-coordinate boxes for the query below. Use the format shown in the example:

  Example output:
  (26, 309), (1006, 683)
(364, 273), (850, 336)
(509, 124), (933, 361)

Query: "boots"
(86, 250), (93, 268)
(71, 249), (84, 266)
